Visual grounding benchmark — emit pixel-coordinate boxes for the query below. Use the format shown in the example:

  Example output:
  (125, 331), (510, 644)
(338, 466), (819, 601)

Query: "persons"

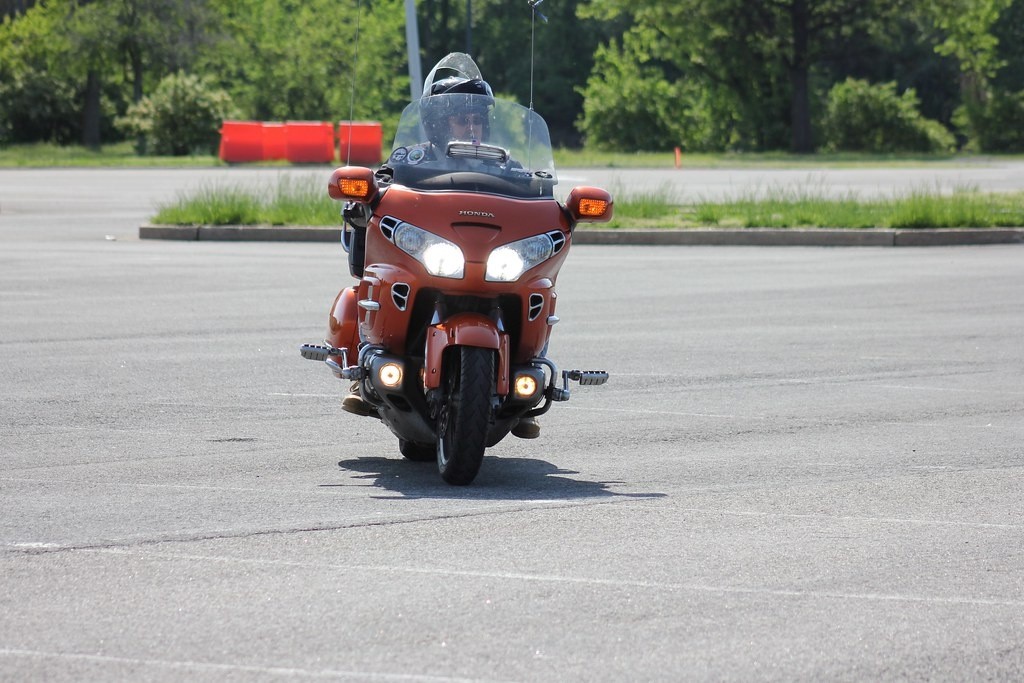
(343, 52), (542, 439)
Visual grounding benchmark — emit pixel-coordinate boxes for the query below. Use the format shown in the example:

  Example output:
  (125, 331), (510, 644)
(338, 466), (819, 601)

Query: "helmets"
(420, 75), (495, 144)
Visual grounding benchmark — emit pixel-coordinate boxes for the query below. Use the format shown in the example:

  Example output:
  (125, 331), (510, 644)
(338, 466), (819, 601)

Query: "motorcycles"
(300, 95), (616, 488)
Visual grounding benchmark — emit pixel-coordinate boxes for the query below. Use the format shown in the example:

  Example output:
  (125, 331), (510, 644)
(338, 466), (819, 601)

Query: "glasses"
(453, 114), (484, 126)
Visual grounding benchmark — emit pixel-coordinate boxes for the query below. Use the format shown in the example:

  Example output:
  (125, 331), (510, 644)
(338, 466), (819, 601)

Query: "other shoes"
(512, 408), (541, 439)
(342, 382), (370, 417)
